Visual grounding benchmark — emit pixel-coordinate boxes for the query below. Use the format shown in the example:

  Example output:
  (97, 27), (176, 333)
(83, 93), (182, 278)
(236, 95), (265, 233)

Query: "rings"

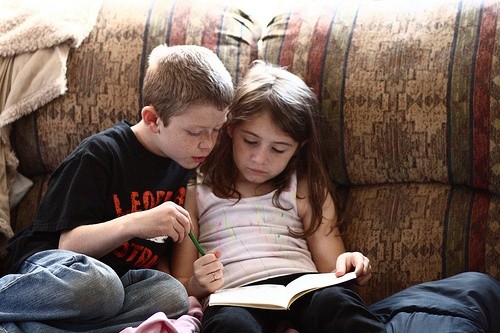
(212, 272), (216, 280)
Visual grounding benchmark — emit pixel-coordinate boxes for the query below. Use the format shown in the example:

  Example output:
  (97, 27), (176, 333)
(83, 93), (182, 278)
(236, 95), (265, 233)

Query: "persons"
(170, 59), (387, 333)
(0, 43), (233, 333)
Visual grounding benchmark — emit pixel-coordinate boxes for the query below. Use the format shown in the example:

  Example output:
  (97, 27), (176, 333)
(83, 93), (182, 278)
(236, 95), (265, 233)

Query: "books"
(208, 272), (357, 311)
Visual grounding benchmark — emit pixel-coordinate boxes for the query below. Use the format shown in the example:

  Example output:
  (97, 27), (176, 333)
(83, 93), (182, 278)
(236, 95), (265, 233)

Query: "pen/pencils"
(188, 231), (205, 256)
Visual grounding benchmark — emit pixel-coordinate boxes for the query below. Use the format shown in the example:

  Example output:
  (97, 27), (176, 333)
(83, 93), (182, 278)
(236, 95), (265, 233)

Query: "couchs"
(0, 0), (499, 307)
